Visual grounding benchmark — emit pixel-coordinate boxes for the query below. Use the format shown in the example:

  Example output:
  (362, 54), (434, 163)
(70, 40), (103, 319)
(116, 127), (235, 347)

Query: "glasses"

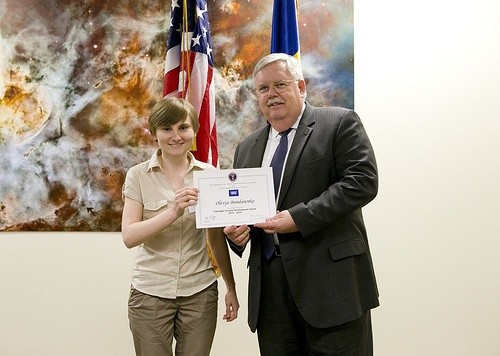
(256, 78), (301, 95)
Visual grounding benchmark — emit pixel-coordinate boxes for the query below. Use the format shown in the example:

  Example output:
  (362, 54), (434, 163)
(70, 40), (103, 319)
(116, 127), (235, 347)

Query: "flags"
(270, 0), (302, 69)
(159, 0), (220, 168)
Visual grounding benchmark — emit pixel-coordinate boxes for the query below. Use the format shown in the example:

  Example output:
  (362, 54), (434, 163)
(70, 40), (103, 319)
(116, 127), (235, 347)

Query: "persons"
(120, 95), (241, 356)
(222, 53), (380, 356)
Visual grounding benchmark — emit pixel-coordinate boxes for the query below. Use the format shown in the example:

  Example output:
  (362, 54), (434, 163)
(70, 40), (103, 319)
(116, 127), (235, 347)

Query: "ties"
(269, 128), (293, 201)
